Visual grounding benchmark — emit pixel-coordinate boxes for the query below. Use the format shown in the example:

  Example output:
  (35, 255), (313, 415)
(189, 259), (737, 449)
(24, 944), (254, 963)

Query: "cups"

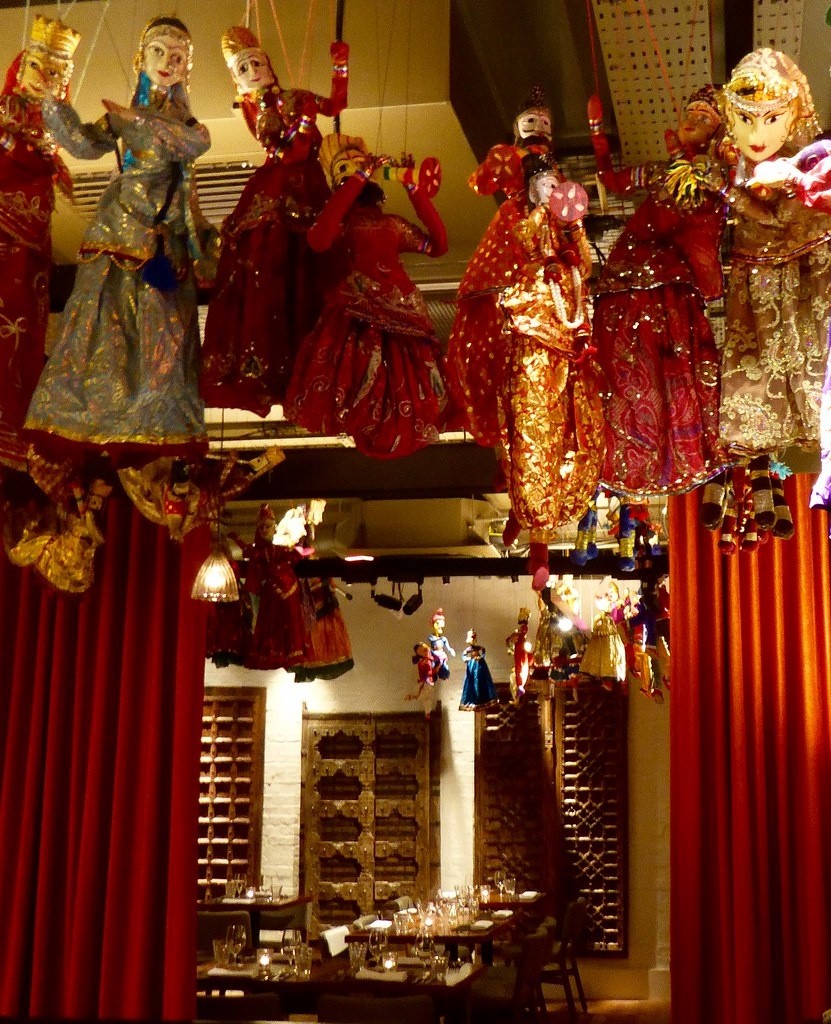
(256, 948), (273, 977)
(245, 887), (255, 899)
(349, 942), (367, 971)
(394, 884), (492, 936)
(225, 882), (235, 898)
(382, 952), (399, 975)
(293, 947), (313, 976)
(430, 950), (450, 981)
(272, 885), (282, 901)
(214, 941), (228, 966)
(505, 878), (516, 895)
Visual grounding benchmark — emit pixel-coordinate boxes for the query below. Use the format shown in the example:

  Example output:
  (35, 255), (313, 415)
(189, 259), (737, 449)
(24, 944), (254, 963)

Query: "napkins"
(355, 968), (407, 982)
(397, 957), (431, 967)
(436, 892), (460, 898)
(491, 910), (513, 918)
(251, 953), (293, 961)
(520, 891), (537, 899)
(397, 908), (417, 915)
(207, 967), (256, 978)
(369, 920), (392, 930)
(243, 892), (272, 896)
(470, 921), (494, 930)
(222, 898), (256, 905)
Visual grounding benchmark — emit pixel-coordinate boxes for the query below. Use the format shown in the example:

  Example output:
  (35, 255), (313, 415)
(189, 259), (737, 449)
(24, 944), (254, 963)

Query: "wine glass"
(415, 934), (435, 978)
(235, 874), (246, 897)
(260, 875), (273, 898)
(281, 930), (301, 965)
(369, 928), (389, 970)
(495, 870), (507, 896)
(228, 929), (247, 966)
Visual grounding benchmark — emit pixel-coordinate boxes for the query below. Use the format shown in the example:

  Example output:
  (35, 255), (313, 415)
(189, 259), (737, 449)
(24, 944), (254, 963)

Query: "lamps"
(370, 576), (424, 615)
(191, 408), (239, 602)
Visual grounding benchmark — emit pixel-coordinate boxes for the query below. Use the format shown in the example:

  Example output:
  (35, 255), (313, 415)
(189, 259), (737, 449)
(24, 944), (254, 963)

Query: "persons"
(0, 14), (831, 719)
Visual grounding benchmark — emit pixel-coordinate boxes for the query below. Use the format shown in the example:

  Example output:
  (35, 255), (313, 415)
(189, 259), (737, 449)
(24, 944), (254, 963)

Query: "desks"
(424, 893), (547, 966)
(196, 956), (314, 998)
(344, 910), (524, 961)
(197, 894), (313, 948)
(269, 958), (486, 1024)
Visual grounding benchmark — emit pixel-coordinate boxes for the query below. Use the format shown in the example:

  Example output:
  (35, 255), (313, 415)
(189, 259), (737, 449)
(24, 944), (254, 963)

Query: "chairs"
(195, 896), (587, 1024)
